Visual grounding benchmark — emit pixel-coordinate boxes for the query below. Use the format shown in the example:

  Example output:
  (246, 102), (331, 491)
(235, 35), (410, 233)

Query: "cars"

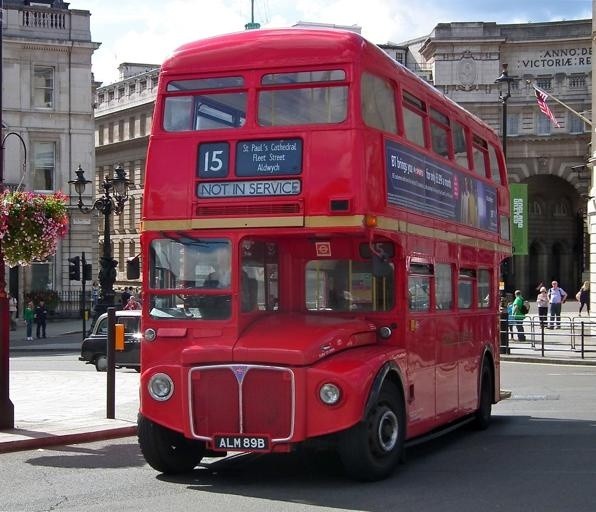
(76, 309), (184, 373)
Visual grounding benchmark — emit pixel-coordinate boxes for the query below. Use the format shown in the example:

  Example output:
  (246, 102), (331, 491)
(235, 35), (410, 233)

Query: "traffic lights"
(66, 256), (79, 280)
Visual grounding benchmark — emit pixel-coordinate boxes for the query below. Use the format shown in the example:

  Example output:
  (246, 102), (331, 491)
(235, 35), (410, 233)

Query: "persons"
(484, 279), (592, 342)
(7, 279), (142, 342)
(182, 247), (250, 319)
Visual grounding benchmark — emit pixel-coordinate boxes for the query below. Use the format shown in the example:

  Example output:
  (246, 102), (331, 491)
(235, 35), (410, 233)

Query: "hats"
(540, 287), (547, 293)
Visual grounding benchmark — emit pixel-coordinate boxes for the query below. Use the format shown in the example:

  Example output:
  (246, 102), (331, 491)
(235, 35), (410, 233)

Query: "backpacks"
(518, 297), (530, 314)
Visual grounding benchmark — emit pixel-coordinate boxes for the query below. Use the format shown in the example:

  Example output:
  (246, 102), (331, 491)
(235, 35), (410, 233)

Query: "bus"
(135, 27), (516, 484)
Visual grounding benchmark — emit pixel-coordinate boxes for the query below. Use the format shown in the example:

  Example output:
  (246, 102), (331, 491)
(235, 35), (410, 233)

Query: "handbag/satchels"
(576, 291), (581, 301)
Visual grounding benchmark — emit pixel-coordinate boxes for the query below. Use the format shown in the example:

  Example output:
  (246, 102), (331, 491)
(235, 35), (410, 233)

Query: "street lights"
(495, 62), (516, 354)
(67, 163), (134, 341)
